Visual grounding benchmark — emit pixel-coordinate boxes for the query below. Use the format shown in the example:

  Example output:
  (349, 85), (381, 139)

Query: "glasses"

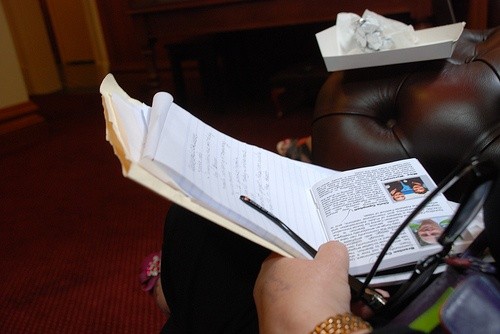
(356, 153), (498, 328)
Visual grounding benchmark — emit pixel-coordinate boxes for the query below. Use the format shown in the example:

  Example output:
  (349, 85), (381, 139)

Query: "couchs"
(313, 26), (500, 201)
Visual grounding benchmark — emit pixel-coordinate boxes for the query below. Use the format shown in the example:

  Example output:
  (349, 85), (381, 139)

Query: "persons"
(140, 157), (500, 334)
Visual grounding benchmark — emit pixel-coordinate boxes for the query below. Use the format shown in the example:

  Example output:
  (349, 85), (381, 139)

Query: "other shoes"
(276, 135), (313, 164)
(138, 249), (171, 314)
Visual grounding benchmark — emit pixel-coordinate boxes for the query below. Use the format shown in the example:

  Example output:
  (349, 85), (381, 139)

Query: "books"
(97, 73), (468, 287)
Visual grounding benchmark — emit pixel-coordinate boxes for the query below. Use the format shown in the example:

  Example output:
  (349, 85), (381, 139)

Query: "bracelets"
(313, 310), (370, 333)
